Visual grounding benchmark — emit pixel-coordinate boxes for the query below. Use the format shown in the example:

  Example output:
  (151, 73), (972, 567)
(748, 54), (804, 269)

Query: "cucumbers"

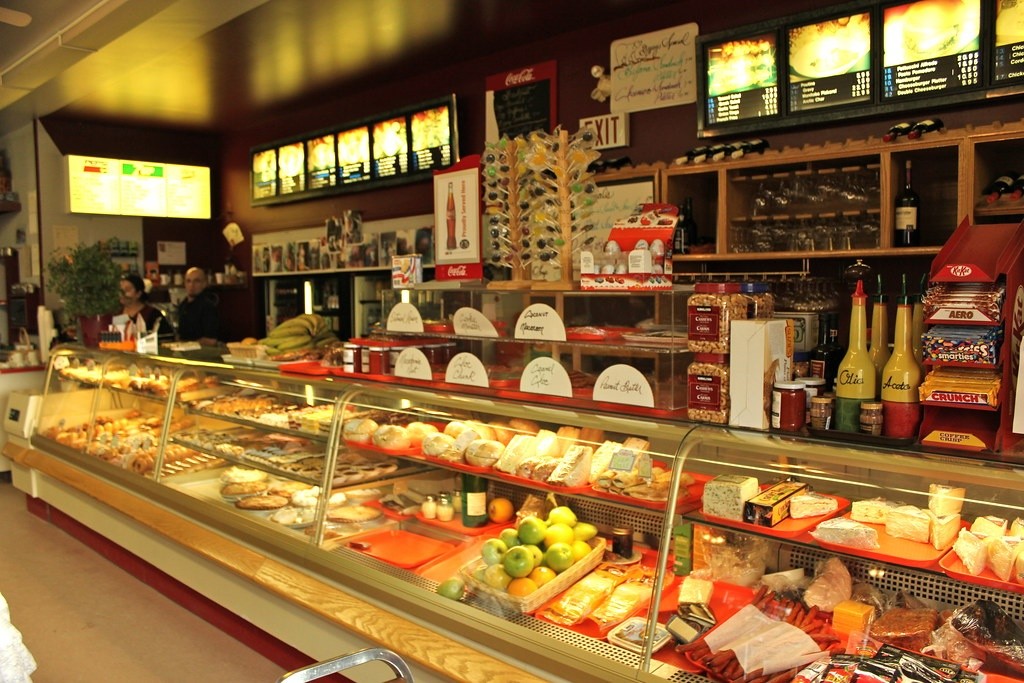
(572, 521), (598, 541)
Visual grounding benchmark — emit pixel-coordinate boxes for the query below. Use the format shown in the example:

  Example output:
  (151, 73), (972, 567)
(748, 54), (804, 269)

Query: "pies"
(218, 482), (383, 529)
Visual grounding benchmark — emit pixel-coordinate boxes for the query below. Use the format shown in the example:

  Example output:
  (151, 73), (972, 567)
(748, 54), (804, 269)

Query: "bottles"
(674, 139), (770, 165)
(462, 472), (488, 526)
(586, 156), (631, 175)
(1009, 173), (1024, 200)
(685, 278), (923, 437)
(437, 496), (454, 521)
(452, 488), (462, 512)
(343, 342), (360, 374)
(421, 494), (437, 519)
(673, 197), (697, 254)
(361, 343), (456, 377)
(437, 490), (452, 504)
(882, 121), (916, 142)
(984, 171), (1018, 203)
(908, 117), (944, 138)
(612, 526), (633, 558)
(446, 182), (457, 249)
(895, 160), (919, 246)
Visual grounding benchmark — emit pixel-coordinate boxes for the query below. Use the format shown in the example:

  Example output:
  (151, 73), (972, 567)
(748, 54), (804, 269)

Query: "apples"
(436, 577), (465, 599)
(472, 507), (590, 600)
(488, 498), (514, 522)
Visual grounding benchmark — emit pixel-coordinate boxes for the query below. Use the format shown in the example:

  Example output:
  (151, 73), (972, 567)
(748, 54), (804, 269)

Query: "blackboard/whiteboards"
(610, 22), (699, 113)
(492, 77), (551, 140)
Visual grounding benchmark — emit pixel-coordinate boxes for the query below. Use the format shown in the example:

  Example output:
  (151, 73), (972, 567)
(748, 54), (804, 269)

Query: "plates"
(342, 420), (1024, 675)
(563, 324), (644, 340)
(221, 354), (332, 376)
(601, 547), (643, 565)
(619, 330), (687, 344)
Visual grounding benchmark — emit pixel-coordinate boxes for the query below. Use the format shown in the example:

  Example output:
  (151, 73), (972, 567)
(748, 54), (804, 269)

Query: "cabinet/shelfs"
(382, 285), (697, 418)
(267, 268), (437, 343)
(915, 213), (1024, 464)
(663, 121), (1024, 261)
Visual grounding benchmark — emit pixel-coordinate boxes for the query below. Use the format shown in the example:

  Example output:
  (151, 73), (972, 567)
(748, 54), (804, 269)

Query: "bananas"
(258, 315), (335, 354)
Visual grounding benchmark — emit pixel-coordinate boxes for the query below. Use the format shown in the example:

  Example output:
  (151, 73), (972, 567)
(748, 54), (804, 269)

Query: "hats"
(121, 273), (149, 300)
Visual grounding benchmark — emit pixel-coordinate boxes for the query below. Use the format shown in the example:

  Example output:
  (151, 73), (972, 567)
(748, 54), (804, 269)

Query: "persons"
(120, 273), (172, 343)
(179, 267), (217, 340)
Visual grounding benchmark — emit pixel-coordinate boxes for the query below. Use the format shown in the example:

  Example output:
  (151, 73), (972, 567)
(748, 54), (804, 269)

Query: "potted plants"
(46, 240), (132, 348)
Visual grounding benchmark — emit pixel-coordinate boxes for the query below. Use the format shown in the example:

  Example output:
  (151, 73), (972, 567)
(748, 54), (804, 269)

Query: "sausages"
(676, 584), (845, 683)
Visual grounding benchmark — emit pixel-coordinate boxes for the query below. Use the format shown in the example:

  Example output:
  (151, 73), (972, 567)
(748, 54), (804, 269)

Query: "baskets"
(227, 341), (266, 359)
(14, 327), (34, 350)
(457, 536), (606, 612)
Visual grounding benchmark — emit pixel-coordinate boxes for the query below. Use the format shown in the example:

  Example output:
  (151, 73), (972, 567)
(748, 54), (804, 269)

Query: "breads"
(676, 576), (714, 604)
(42, 362), (388, 480)
(343, 419), (696, 505)
(704, 474), (1024, 673)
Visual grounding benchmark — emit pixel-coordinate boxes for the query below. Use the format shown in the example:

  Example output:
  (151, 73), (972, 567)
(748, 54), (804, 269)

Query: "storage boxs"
(98, 312), (134, 354)
(730, 318), (795, 431)
(135, 318), (158, 355)
(773, 310), (819, 354)
(577, 200), (681, 293)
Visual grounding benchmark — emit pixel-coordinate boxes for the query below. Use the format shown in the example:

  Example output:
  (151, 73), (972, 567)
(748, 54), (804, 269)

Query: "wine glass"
(729, 165), (880, 251)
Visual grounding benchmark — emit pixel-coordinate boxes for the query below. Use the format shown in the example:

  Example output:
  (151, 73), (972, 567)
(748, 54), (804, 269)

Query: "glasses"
(185, 279), (201, 283)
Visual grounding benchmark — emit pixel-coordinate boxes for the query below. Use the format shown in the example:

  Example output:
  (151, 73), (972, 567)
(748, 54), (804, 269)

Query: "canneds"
(686, 280), (884, 437)
(343, 342), (456, 374)
(611, 525), (634, 560)
